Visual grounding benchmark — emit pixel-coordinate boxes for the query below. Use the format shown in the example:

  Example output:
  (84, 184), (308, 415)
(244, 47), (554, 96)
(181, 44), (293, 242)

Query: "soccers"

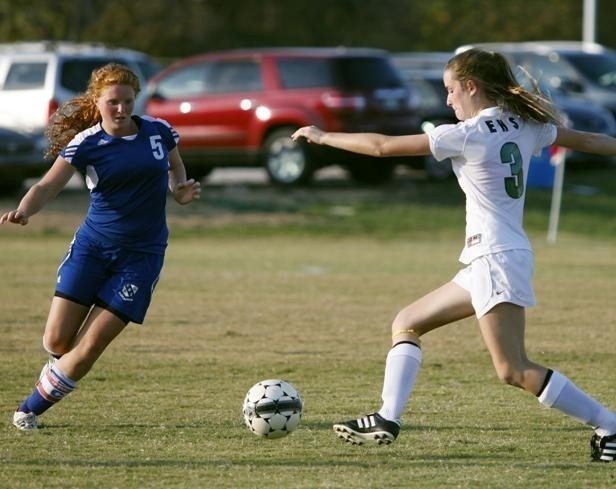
(243, 380), (302, 438)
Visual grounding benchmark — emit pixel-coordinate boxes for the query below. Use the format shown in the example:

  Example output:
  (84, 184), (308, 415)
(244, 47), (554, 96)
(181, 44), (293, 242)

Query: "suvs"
(0, 40), (161, 201)
(139, 45), (418, 186)
(388, 35), (616, 190)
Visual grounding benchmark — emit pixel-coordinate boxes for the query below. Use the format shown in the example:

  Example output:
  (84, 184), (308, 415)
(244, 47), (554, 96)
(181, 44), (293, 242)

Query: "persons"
(0, 63), (202, 433)
(289, 47), (616, 465)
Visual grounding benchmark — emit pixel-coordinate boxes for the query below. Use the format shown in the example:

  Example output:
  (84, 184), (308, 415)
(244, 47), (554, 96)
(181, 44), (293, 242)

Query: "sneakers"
(13, 406), (39, 431)
(333, 412), (401, 447)
(40, 353), (61, 379)
(589, 431), (615, 462)
